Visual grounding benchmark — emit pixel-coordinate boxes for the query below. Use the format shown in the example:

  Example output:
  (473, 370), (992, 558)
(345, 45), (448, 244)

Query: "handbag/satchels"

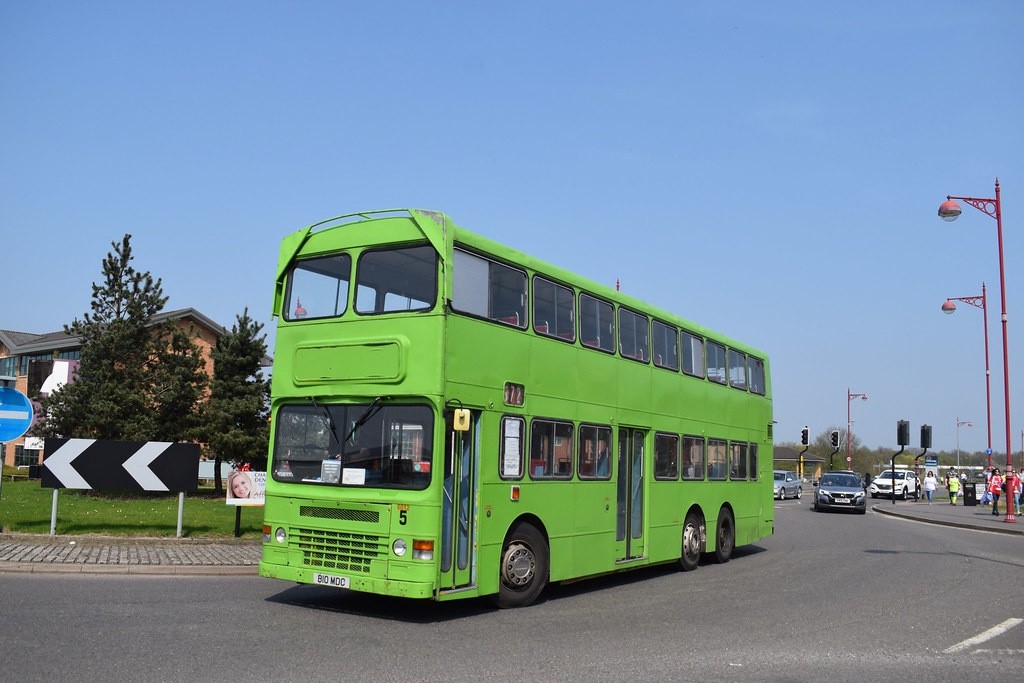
(1017, 481), (1023, 494)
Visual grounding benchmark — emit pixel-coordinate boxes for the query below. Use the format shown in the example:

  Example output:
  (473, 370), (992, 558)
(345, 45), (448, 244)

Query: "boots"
(950, 496), (953, 504)
(953, 497), (957, 506)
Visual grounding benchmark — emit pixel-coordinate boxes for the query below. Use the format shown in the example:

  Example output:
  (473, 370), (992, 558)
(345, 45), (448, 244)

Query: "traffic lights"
(896, 419), (910, 445)
(800, 426), (810, 446)
(920, 424), (933, 448)
(831, 430), (840, 448)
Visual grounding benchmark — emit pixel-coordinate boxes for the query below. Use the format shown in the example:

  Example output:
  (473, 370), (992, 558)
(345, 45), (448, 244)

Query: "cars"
(813, 472), (867, 514)
(774, 470), (803, 501)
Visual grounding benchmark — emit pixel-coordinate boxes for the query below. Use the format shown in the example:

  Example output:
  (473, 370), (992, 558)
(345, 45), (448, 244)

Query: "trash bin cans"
(962, 482), (977, 506)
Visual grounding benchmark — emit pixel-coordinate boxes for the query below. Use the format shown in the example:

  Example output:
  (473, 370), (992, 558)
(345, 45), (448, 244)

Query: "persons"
(944, 467), (968, 505)
(229, 472), (252, 498)
(923, 471), (937, 504)
(984, 466), (1024, 516)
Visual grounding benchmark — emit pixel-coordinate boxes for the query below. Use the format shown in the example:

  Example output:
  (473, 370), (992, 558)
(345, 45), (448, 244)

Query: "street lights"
(941, 279), (993, 470)
(955, 417), (973, 473)
(938, 177), (1016, 524)
(847, 387), (867, 470)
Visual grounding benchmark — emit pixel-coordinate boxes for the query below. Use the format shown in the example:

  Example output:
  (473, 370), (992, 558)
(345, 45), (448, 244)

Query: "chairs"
(707, 371), (758, 393)
(558, 330), (663, 365)
(535, 320), (548, 332)
(558, 457), (726, 477)
(492, 310), (519, 326)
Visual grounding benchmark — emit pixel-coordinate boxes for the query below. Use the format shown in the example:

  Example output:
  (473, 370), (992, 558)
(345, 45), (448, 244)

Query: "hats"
(952, 472), (957, 475)
(950, 466), (955, 470)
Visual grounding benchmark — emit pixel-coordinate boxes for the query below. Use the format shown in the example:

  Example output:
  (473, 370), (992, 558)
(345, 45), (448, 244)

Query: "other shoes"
(992, 512), (996, 515)
(930, 503), (932, 505)
(1018, 512), (1022, 516)
(996, 513), (999, 516)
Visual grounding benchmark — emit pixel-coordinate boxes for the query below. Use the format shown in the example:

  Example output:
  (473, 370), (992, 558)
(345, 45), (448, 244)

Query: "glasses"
(995, 471), (999, 472)
(928, 474), (932, 475)
(1012, 471), (1017, 473)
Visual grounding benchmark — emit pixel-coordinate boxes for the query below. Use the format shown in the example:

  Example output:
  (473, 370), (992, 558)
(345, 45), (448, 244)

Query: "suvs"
(870, 469), (922, 501)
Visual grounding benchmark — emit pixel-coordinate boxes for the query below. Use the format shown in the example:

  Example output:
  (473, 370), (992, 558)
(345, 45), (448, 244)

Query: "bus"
(259, 207), (777, 608)
